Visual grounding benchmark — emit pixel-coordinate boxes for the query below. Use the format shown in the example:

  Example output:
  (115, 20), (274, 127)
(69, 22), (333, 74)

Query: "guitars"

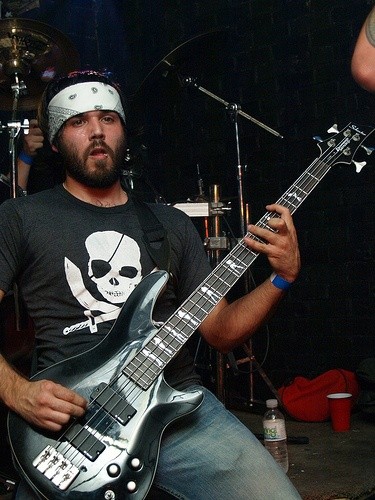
(5, 121), (374, 500)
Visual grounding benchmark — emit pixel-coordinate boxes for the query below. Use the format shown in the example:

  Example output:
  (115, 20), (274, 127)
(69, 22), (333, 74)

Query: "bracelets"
(18, 151), (35, 165)
(270, 271), (293, 290)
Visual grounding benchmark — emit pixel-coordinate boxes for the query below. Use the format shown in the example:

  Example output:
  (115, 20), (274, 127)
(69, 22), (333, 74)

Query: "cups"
(326, 393), (353, 432)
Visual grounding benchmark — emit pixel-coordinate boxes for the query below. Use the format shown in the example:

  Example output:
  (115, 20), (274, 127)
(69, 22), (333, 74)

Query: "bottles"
(262, 399), (289, 474)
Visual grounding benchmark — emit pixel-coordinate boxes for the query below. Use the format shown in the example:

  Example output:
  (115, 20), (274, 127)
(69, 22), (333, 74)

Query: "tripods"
(186, 183), (282, 411)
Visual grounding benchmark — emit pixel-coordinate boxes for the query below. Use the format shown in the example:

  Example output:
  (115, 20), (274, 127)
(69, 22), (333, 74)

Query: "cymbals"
(0, 17), (81, 113)
(130, 27), (229, 111)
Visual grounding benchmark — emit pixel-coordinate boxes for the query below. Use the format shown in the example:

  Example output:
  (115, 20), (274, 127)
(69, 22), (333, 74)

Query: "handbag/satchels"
(278, 370), (356, 423)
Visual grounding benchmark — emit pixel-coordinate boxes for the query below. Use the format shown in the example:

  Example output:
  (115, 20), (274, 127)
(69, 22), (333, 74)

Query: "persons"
(0, 110), (67, 205)
(351, 5), (375, 93)
(0, 71), (300, 500)
